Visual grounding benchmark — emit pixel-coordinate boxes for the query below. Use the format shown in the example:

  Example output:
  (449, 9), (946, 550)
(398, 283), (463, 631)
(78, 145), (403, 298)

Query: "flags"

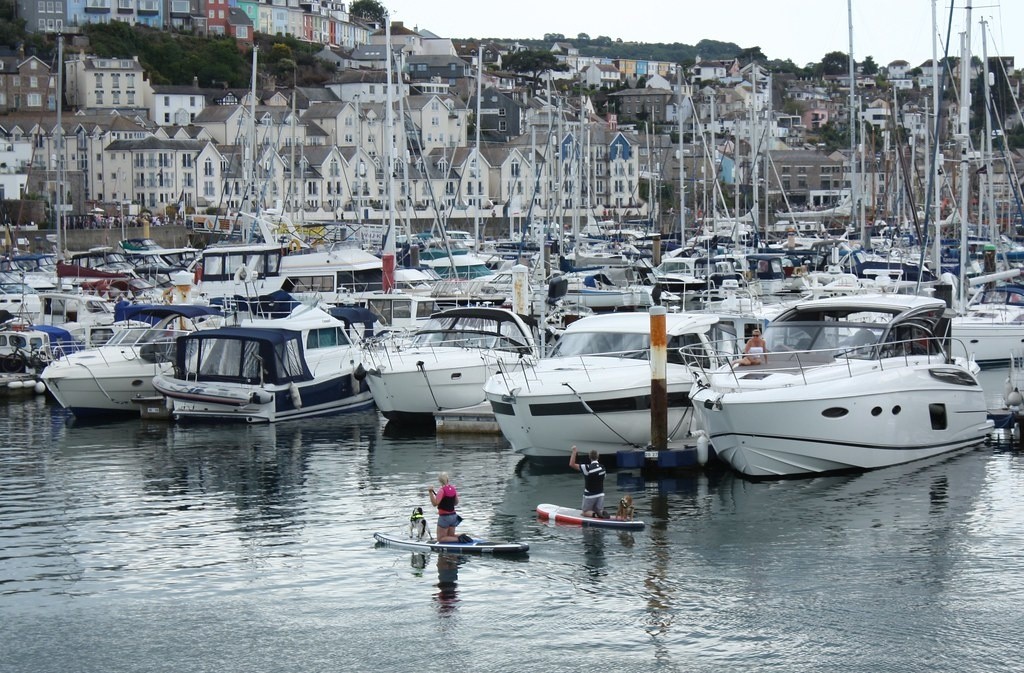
(718, 154), (734, 184)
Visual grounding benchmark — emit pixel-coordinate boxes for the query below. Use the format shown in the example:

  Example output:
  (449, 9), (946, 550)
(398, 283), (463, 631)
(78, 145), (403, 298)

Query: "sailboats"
(0, 1), (1024, 479)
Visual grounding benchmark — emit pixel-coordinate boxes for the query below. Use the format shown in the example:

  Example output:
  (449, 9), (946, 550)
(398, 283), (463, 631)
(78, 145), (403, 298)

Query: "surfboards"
(537, 503), (645, 530)
(373, 532), (530, 554)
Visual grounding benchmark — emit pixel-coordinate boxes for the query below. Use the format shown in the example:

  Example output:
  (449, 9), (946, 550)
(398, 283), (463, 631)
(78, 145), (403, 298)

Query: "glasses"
(752, 334), (760, 337)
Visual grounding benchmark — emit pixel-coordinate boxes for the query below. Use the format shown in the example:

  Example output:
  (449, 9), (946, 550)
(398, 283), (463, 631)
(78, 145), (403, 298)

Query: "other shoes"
(596, 510), (610, 519)
(458, 533), (473, 542)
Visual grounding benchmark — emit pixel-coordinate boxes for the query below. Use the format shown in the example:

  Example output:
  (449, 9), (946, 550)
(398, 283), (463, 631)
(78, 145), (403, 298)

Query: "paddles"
(576, 452), (582, 464)
(432, 489), (436, 496)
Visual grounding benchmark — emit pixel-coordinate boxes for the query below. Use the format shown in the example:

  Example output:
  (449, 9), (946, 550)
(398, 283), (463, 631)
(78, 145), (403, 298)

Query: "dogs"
(615, 495), (634, 521)
(409, 506), (432, 540)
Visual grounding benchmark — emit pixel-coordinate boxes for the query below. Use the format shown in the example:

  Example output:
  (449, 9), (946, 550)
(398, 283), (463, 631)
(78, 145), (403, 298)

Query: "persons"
(906, 330), (929, 351)
(24, 235), (30, 253)
(569, 445), (610, 519)
(96, 212), (192, 227)
(1011, 293), (1019, 301)
(854, 321), (877, 355)
(729, 329), (768, 371)
(429, 474), (473, 543)
